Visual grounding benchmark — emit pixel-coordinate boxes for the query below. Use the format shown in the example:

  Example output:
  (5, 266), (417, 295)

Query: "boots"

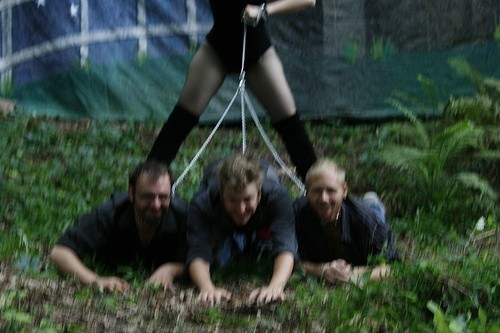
(148, 101), (200, 167)
(270, 110), (318, 184)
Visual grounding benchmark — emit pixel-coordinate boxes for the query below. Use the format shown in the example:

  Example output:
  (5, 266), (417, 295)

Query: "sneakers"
(363, 192), (386, 214)
